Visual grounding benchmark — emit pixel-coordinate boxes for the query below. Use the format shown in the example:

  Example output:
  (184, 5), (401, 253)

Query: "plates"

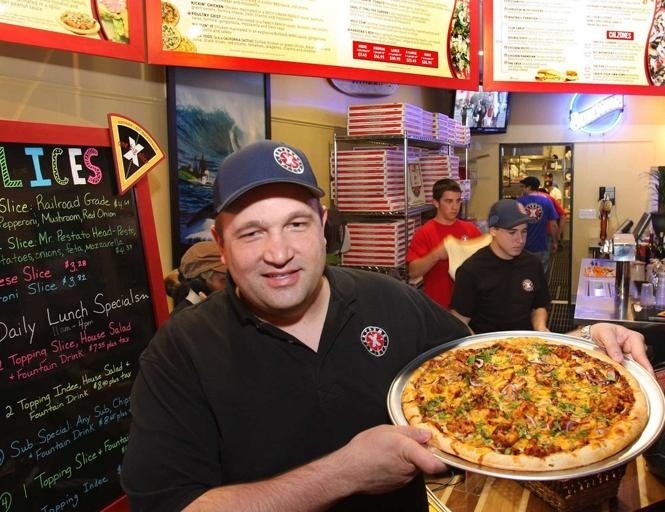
(388, 328), (665, 481)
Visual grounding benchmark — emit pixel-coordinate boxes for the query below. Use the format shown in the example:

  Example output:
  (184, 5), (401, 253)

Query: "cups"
(640, 271), (665, 310)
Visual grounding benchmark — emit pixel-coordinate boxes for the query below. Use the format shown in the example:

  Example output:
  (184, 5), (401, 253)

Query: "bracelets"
(574, 322), (594, 343)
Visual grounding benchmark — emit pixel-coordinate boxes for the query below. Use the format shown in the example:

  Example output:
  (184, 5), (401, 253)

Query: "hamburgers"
(565, 71), (578, 82)
(535, 69), (561, 82)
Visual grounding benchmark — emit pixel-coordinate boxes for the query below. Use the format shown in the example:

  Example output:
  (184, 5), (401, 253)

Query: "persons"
(449, 197), (553, 338)
(406, 179), (485, 307)
(116, 141), (658, 512)
(542, 171), (566, 213)
(514, 174), (558, 278)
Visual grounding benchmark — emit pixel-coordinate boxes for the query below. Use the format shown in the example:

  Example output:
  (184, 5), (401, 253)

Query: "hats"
(180, 242), (227, 279)
(520, 177), (539, 189)
(215, 140), (325, 213)
(542, 173), (552, 178)
(488, 199), (535, 230)
(544, 181), (552, 187)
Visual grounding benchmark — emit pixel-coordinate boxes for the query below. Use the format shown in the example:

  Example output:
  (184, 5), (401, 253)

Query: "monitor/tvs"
(450, 80), (512, 133)
(633, 212), (652, 238)
(609, 218), (633, 244)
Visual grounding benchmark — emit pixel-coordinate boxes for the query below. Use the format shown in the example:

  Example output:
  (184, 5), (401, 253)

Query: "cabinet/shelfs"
(330, 132), (468, 292)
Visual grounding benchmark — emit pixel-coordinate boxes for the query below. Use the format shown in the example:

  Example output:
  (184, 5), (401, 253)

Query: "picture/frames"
(165, 60), (272, 271)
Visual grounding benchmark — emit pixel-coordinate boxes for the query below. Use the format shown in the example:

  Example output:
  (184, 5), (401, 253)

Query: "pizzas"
(400, 337), (649, 473)
(63, 8), (96, 30)
(162, 2), (197, 52)
